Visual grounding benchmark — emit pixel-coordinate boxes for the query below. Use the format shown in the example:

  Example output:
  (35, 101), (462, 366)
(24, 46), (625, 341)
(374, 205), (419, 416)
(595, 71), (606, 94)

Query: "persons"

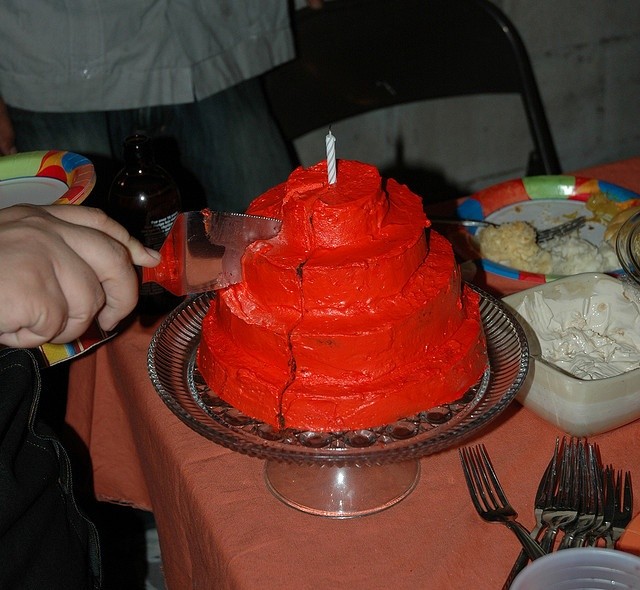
(1, 199), (161, 589)
(1, 2), (305, 260)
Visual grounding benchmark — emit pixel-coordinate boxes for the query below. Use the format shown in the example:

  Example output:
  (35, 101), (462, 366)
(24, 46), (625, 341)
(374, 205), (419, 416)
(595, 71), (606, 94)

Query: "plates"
(454, 174), (640, 283)
(0, 149), (97, 210)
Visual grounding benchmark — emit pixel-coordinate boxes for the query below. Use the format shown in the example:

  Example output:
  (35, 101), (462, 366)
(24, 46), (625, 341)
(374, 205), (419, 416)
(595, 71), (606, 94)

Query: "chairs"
(262, 0), (562, 174)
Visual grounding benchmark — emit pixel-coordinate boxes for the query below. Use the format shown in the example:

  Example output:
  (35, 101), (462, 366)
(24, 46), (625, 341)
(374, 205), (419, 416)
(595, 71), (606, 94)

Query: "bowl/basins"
(509, 547), (640, 590)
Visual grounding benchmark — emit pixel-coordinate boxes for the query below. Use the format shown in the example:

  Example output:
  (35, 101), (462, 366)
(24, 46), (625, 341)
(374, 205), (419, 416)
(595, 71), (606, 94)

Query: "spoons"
(502, 437), (631, 590)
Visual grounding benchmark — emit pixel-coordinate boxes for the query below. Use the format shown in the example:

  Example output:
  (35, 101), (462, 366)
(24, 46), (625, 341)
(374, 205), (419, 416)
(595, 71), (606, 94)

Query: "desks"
(63, 156), (639, 589)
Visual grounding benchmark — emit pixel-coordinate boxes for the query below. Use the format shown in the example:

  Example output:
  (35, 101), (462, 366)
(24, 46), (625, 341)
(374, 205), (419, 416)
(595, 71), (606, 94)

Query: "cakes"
(195, 124), (487, 432)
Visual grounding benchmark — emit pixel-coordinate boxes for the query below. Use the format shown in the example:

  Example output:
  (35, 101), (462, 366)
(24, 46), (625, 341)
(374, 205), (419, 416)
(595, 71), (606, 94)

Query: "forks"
(425, 217), (586, 244)
(454, 443), (546, 558)
